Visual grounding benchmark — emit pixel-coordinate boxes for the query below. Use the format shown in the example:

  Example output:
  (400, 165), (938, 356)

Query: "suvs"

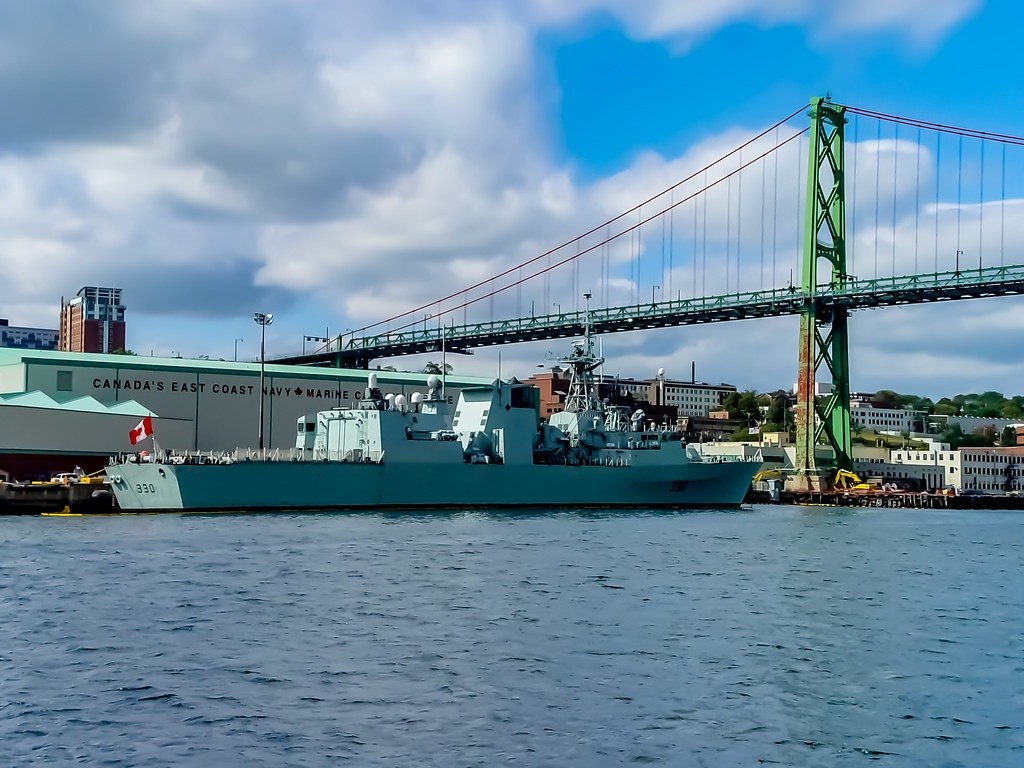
(50, 473), (80, 482)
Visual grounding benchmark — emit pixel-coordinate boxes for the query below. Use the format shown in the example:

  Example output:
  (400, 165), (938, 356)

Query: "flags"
(129, 416), (153, 445)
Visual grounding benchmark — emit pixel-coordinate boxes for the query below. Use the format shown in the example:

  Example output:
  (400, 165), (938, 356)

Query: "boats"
(41, 506), (83, 517)
(103, 294), (766, 507)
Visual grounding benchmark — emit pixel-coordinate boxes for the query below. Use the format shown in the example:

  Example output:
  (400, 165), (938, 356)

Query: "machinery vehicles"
(832, 468), (876, 494)
